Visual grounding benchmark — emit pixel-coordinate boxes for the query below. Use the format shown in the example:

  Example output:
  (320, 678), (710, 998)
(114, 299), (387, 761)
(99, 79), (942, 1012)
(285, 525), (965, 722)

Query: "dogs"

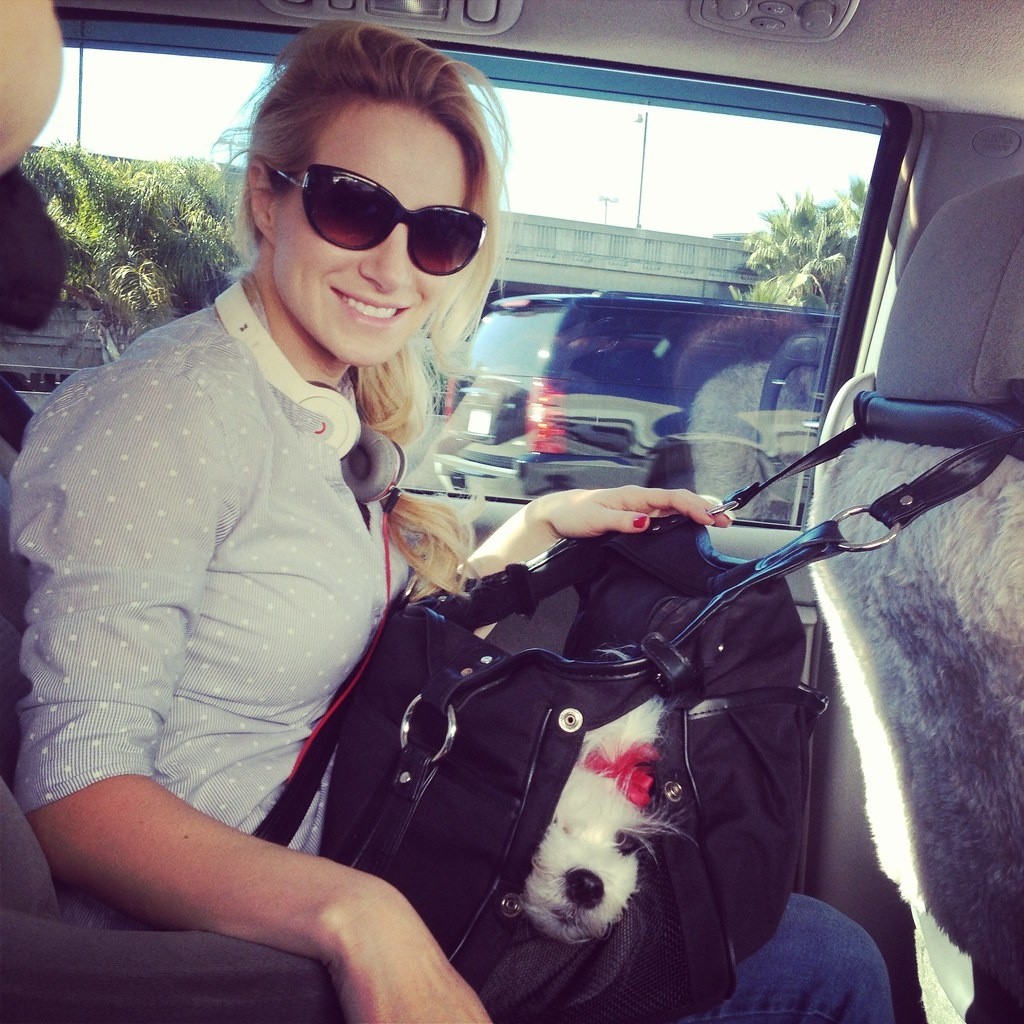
(521, 689), (671, 948)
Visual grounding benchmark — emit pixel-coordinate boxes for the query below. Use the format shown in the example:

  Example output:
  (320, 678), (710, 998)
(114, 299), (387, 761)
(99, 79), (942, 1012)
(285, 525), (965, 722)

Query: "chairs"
(803, 173), (1024, 1024)
(0, 381), (351, 1024)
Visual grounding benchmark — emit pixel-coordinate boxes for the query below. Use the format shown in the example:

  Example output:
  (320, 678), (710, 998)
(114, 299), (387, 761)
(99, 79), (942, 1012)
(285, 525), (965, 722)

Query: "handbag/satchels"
(267, 405), (1007, 1024)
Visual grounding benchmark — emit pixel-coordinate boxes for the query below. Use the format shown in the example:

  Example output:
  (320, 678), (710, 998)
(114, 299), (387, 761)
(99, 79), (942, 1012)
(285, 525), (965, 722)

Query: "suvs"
(435, 289), (836, 526)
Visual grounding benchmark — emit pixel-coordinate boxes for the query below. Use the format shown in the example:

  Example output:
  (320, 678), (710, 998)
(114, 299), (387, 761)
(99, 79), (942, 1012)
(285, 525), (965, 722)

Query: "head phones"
(215, 281), (408, 504)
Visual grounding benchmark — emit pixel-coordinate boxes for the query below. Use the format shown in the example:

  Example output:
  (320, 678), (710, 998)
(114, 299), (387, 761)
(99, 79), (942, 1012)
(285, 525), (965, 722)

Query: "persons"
(0, 25), (904, 1024)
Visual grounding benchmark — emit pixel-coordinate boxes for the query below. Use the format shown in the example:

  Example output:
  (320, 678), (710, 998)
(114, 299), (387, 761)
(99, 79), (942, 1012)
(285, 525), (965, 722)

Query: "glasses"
(268, 159), (487, 277)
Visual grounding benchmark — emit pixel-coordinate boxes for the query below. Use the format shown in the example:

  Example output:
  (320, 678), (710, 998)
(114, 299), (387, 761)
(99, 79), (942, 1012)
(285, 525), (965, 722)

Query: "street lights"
(600, 195), (616, 225)
(633, 112), (649, 225)
